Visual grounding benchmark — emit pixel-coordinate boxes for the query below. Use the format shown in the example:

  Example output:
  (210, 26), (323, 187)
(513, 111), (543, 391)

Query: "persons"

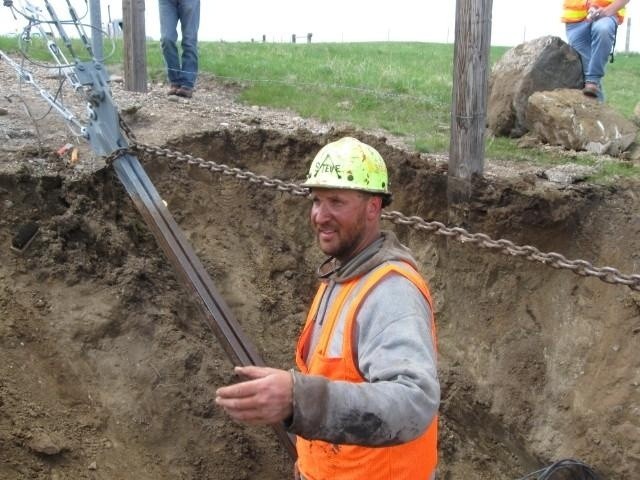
(158, 0), (200, 97)
(213, 135), (441, 480)
(561, 0), (630, 97)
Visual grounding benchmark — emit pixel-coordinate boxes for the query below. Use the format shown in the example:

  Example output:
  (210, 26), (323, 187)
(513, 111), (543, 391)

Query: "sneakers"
(167, 86), (192, 98)
(582, 81), (600, 97)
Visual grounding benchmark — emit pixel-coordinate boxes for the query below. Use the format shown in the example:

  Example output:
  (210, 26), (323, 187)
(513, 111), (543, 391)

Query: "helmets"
(299, 137), (393, 198)
